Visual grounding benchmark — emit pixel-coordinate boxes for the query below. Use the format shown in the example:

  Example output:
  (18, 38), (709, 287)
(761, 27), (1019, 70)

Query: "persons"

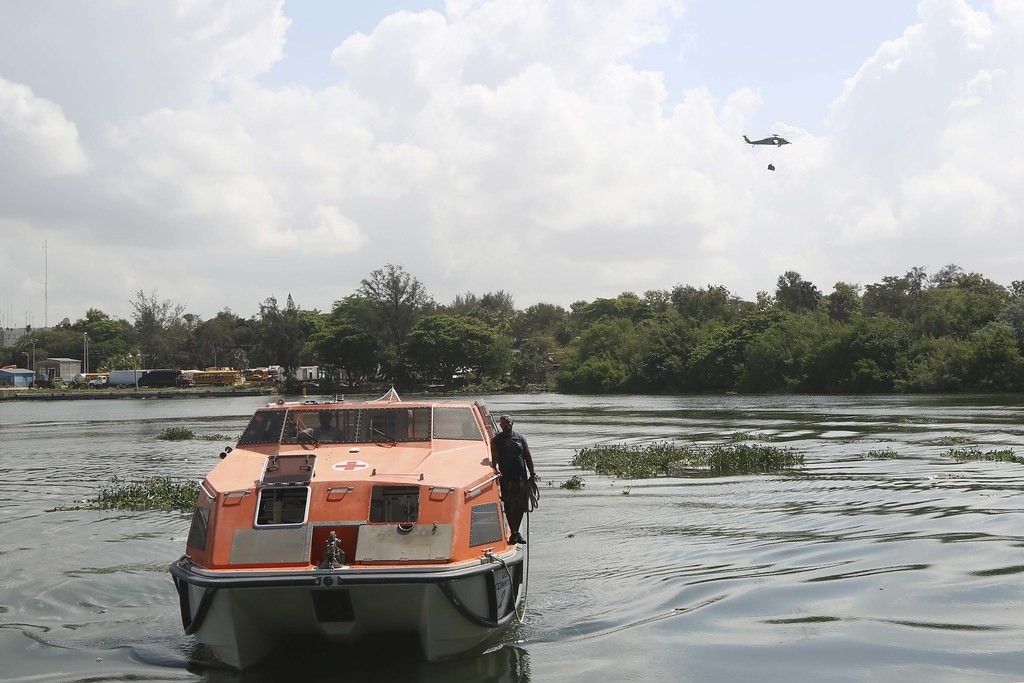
(492, 415), (535, 545)
(297, 412), (344, 441)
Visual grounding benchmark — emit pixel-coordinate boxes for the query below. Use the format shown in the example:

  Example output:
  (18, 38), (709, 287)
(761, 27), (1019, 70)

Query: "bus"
(193, 370), (241, 387)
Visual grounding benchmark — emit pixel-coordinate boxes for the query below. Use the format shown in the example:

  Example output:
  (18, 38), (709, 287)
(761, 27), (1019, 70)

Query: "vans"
(138, 370), (182, 388)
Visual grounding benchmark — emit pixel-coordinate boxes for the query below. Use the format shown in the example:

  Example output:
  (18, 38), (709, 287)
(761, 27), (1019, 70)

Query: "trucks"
(68, 372), (110, 389)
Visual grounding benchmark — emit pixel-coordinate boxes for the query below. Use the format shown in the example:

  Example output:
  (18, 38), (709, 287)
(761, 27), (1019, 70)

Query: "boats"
(170, 387), (523, 670)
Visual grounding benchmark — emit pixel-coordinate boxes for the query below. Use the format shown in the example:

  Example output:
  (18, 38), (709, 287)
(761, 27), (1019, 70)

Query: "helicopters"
(743, 134), (792, 148)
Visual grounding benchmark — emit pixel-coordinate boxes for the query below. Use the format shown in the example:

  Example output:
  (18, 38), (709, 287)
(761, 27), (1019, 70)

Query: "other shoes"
(509, 532), (519, 544)
(518, 533), (526, 544)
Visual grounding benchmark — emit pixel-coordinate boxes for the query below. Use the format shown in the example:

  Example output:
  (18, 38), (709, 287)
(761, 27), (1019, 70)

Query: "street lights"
(22, 352), (29, 370)
(128, 352), (142, 390)
